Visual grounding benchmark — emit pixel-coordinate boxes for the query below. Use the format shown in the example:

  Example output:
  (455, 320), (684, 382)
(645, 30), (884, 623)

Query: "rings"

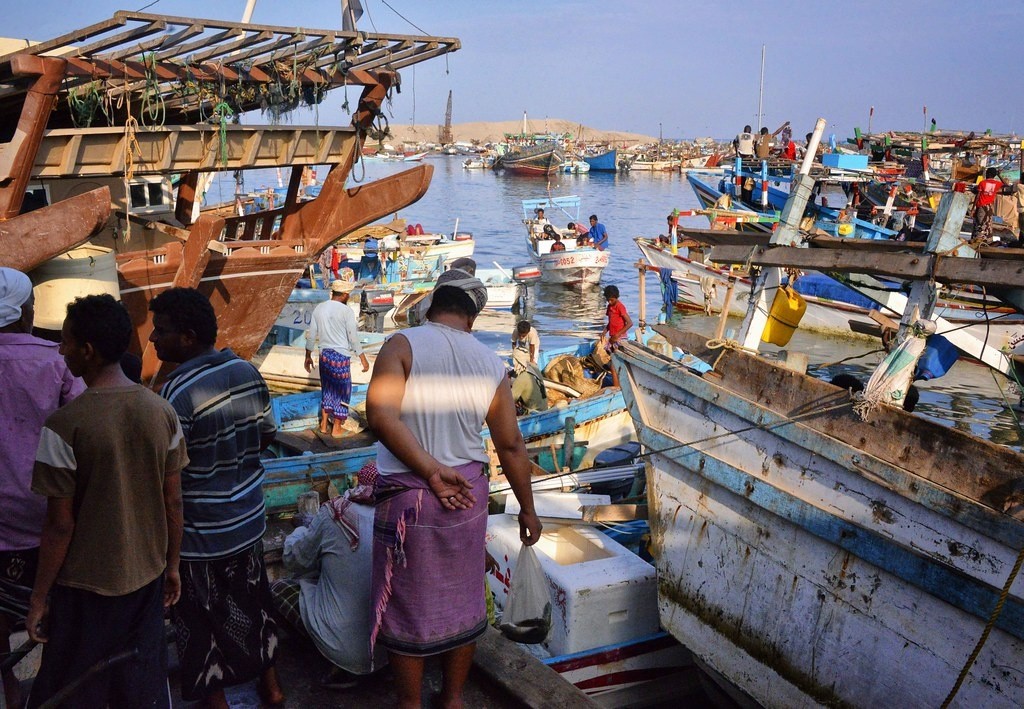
(449, 497), (454, 501)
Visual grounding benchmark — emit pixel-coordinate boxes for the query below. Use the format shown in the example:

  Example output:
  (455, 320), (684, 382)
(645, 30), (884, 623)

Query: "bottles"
(915, 334), (958, 383)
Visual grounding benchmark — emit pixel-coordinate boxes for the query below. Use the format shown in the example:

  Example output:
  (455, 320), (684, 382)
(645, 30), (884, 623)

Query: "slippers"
(317, 424), (329, 434)
(333, 430), (356, 439)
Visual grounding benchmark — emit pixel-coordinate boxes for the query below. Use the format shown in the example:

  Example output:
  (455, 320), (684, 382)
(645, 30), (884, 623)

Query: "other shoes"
(258, 672), (286, 705)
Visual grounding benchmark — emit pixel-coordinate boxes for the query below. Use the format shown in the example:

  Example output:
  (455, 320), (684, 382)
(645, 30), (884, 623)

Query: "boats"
(206, 105), (1024, 698)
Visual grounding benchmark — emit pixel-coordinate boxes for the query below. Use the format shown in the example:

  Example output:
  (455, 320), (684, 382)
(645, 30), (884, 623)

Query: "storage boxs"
(822, 153), (868, 169)
(486, 510), (659, 660)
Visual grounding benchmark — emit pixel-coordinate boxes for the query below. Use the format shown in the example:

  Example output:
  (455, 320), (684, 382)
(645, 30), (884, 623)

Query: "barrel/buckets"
(761, 287), (807, 347)
(839, 224), (852, 234)
(29, 245), (121, 331)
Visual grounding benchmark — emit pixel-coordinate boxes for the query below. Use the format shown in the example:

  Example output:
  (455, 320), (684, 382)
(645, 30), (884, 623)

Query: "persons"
(146, 285), (285, 709)
(733, 125), (824, 161)
(510, 348), (548, 415)
(600, 285), (633, 390)
(267, 460), (388, 679)
(303, 280), (370, 438)
(0, 267), (88, 709)
(970, 168), (1007, 244)
(584, 215), (608, 249)
(659, 215), (684, 245)
(550, 234), (565, 251)
(26, 293), (193, 709)
(365, 270), (543, 709)
(530, 208), (556, 235)
(568, 222), (590, 243)
(511, 321), (540, 365)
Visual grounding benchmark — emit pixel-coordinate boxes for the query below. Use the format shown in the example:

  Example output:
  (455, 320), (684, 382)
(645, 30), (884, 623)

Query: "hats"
(435, 270), (488, 312)
(0, 267), (33, 326)
(332, 280), (354, 293)
